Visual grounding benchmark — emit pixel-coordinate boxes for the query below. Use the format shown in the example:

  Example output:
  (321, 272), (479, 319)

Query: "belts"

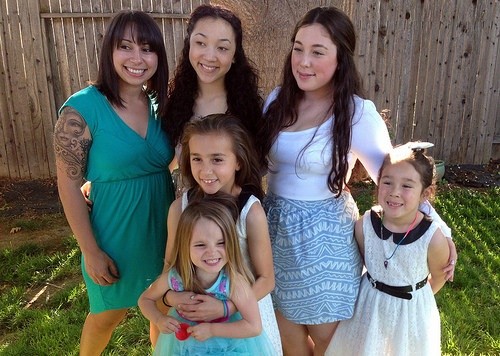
(367, 271), (428, 300)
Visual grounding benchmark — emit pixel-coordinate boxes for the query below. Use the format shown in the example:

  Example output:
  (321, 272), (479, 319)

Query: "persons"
(137, 201), (285, 356)
(262, 6), (459, 356)
(150, 113), (285, 356)
(324, 145), (451, 356)
(53, 12), (178, 356)
(81, 3), (270, 240)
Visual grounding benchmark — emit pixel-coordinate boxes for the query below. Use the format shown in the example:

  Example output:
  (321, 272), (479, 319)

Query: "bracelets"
(222, 300), (230, 319)
(162, 288), (175, 308)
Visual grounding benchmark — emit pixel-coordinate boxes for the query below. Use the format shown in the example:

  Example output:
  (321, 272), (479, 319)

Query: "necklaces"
(380, 209), (419, 269)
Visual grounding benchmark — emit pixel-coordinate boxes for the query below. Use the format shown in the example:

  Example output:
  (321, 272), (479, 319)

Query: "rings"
(191, 295), (194, 298)
(450, 260), (454, 265)
